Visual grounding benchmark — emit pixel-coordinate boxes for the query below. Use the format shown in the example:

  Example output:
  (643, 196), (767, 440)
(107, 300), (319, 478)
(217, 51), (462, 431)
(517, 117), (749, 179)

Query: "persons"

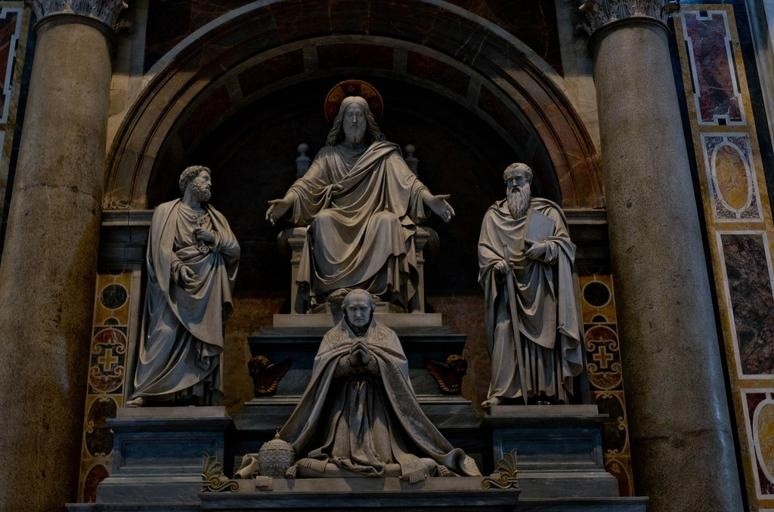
(475, 161), (585, 406)
(125, 166), (243, 406)
(266, 95), (457, 312)
(233, 287), (483, 484)
